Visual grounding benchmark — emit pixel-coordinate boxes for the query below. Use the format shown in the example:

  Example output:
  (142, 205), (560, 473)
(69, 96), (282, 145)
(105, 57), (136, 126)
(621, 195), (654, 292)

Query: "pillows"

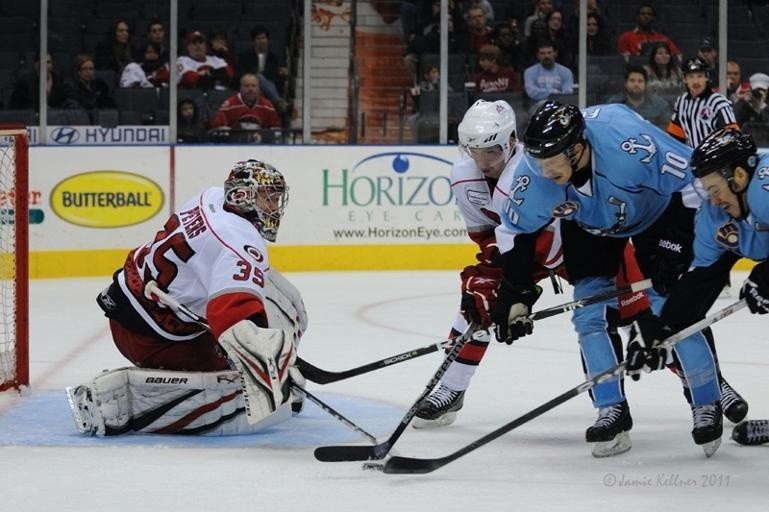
(458, 99), (519, 163)
(522, 101), (586, 157)
(222, 159), (289, 243)
(690, 126), (758, 178)
(683, 56), (709, 74)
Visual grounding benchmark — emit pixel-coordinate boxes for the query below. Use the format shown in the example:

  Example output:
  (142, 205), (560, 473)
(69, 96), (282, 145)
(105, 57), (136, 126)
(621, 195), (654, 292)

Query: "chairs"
(691, 401), (723, 443)
(1, 1), (305, 145)
(416, 386), (464, 420)
(585, 402), (632, 441)
(721, 377), (748, 423)
(409, 0), (768, 151)
(732, 419), (769, 445)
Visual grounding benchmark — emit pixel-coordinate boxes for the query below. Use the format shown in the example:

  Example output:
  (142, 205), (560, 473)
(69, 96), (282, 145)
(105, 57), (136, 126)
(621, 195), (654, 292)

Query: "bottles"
(188, 31), (206, 44)
(698, 36), (714, 49)
(749, 73), (768, 90)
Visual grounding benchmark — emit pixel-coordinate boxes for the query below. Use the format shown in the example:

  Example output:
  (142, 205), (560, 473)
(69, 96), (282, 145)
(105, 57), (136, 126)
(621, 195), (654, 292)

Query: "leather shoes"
(739, 262), (769, 315)
(495, 287), (534, 345)
(625, 316), (674, 381)
(460, 264), (502, 327)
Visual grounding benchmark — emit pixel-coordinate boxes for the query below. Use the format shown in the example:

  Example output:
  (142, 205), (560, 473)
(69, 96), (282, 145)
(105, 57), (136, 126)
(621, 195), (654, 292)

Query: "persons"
(697, 36), (718, 71)
(665, 58), (744, 149)
(412, 97), (750, 428)
(468, 46), (522, 91)
(487, 98), (723, 457)
(176, 99), (207, 133)
(416, 65), (452, 92)
(214, 71), (283, 129)
(147, 31), (235, 84)
(603, 65), (673, 132)
(63, 54), (117, 107)
(733, 71), (769, 145)
(641, 124), (768, 443)
(524, 44), (577, 100)
(644, 42), (687, 87)
(208, 28), (235, 63)
(241, 27), (293, 115)
(98, 22), (142, 66)
(145, 25), (166, 51)
(117, 40), (172, 89)
(6, 47), (65, 111)
(67, 159), (308, 435)
(712, 60), (750, 100)
(413, 2), (686, 55)
